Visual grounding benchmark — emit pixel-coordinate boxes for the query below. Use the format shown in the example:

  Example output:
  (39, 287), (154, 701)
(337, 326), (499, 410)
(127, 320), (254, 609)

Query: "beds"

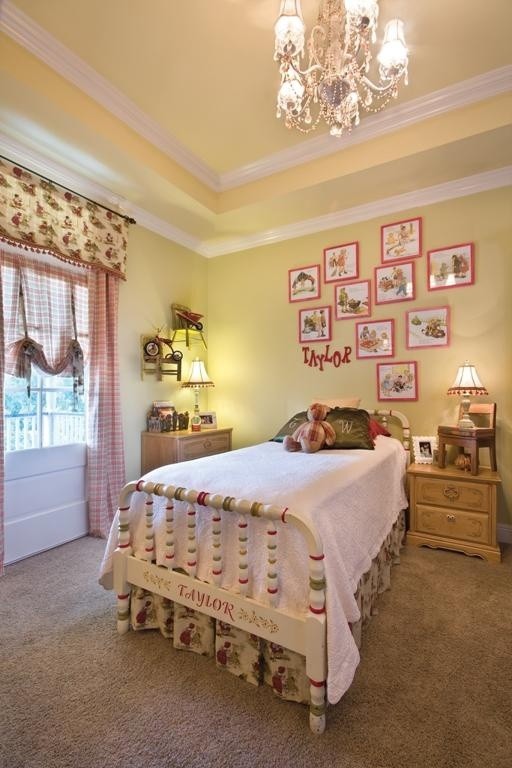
(98, 409), (411, 735)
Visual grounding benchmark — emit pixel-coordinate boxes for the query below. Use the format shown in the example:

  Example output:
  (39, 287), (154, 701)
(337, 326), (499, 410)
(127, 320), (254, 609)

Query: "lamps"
(180, 358), (214, 416)
(269, 0), (411, 140)
(444, 359), (488, 428)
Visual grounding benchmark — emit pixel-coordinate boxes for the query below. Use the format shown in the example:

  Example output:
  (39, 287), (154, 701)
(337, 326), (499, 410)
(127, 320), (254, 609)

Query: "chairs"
(169, 302), (209, 351)
(139, 332), (182, 382)
(438, 402), (496, 474)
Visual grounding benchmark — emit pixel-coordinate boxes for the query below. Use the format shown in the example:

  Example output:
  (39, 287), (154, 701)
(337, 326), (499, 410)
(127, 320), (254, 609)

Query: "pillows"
(368, 418), (390, 440)
(269, 407), (375, 451)
(310, 396), (363, 408)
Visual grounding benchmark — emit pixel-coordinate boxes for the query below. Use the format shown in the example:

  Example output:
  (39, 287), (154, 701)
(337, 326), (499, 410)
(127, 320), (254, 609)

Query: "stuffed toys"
(282, 402), (337, 454)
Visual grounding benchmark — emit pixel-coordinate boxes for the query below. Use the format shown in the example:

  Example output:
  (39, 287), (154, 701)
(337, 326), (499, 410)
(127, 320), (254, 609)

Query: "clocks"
(144, 341), (161, 356)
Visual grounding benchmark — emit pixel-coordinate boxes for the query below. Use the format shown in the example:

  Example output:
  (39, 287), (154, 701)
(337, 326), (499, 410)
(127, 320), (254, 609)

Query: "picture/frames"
(286, 263), (321, 303)
(411, 436), (436, 465)
(407, 305), (449, 350)
(194, 410), (217, 429)
(299, 305), (331, 341)
(375, 360), (419, 402)
(425, 242), (474, 292)
(334, 280), (372, 321)
(373, 261), (415, 306)
(355, 319), (394, 359)
(379, 216), (422, 264)
(322, 241), (360, 283)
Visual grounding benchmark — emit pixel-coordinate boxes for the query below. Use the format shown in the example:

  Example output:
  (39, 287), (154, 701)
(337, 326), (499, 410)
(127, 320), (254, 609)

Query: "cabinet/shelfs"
(140, 425), (234, 477)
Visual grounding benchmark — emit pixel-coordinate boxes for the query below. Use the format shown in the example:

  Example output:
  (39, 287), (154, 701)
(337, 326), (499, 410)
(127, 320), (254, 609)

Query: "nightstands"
(403, 459), (503, 563)
